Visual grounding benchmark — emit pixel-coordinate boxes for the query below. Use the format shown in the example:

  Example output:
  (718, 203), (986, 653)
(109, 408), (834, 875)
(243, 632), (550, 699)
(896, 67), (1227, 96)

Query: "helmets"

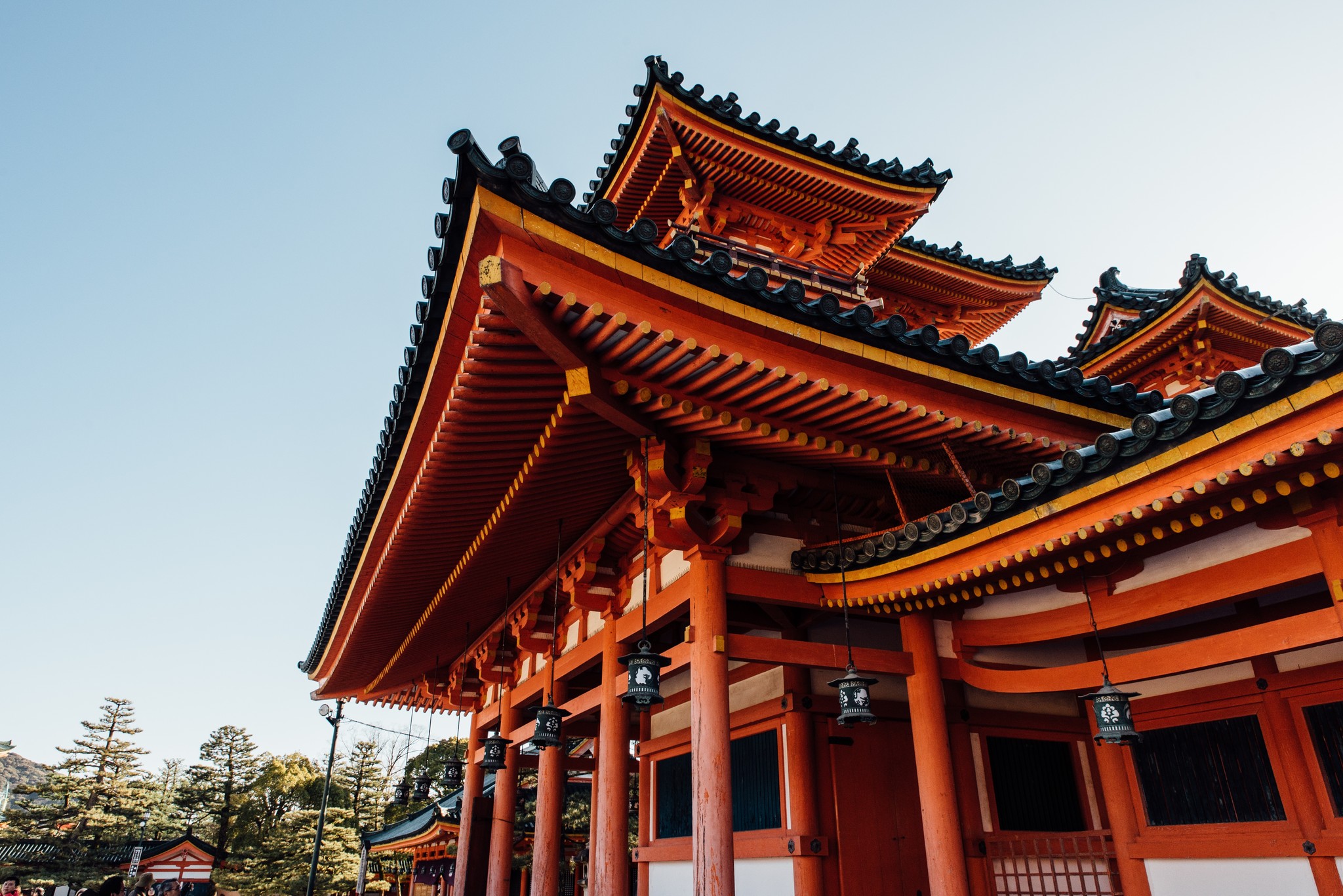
(75, 888), (88, 896)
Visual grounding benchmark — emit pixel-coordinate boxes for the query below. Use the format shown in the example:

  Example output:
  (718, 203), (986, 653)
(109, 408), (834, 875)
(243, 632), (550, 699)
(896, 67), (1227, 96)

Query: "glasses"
(165, 886), (181, 891)
(120, 889), (127, 893)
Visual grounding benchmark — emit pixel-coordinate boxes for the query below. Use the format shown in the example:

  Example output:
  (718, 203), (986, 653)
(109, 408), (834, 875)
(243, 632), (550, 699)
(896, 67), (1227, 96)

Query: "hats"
(135, 872), (157, 887)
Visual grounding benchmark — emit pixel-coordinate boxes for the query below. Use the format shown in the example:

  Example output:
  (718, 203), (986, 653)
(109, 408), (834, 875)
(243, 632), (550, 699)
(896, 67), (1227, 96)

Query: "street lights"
(122, 808), (152, 896)
(304, 697), (346, 896)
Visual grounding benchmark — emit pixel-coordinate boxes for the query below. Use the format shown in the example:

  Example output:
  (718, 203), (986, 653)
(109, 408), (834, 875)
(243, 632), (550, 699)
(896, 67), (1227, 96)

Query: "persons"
(0, 871), (219, 896)
